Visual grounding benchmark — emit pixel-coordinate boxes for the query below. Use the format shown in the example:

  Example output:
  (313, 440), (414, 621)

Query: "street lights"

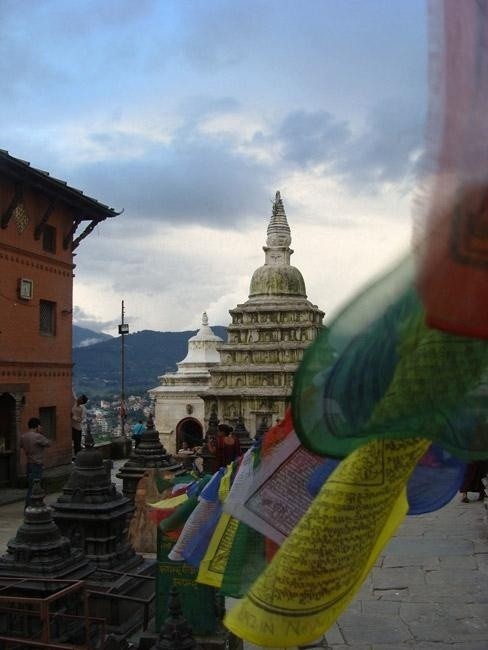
(119, 300), (129, 436)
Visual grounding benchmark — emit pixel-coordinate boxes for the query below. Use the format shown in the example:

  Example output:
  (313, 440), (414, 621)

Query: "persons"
(180, 441), (190, 452)
(71, 382), (90, 457)
(459, 463), (486, 503)
(207, 423), (242, 474)
(20, 418), (53, 516)
(132, 419), (146, 449)
